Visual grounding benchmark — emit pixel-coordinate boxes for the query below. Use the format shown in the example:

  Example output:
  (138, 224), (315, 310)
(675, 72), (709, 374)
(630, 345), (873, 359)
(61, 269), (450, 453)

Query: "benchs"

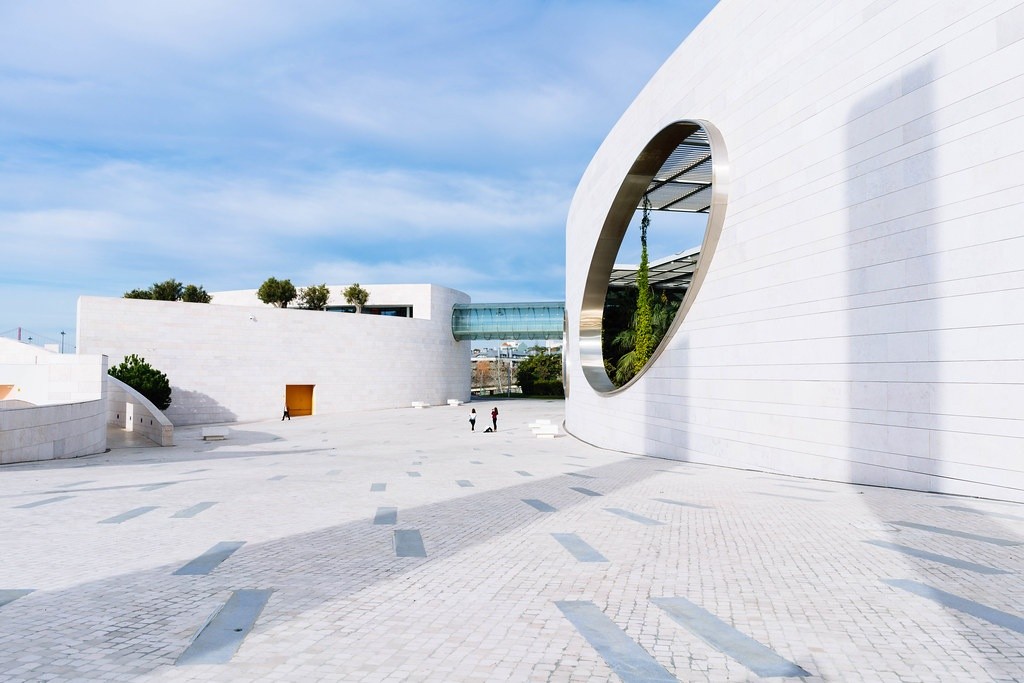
(202, 427), (229, 441)
(411, 401), (430, 409)
(447, 399), (463, 406)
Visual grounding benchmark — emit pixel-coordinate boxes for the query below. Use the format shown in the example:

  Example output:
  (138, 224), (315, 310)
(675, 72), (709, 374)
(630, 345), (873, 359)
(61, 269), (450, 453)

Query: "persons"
(492, 407), (498, 430)
(469, 409), (477, 432)
(281, 403), (290, 421)
